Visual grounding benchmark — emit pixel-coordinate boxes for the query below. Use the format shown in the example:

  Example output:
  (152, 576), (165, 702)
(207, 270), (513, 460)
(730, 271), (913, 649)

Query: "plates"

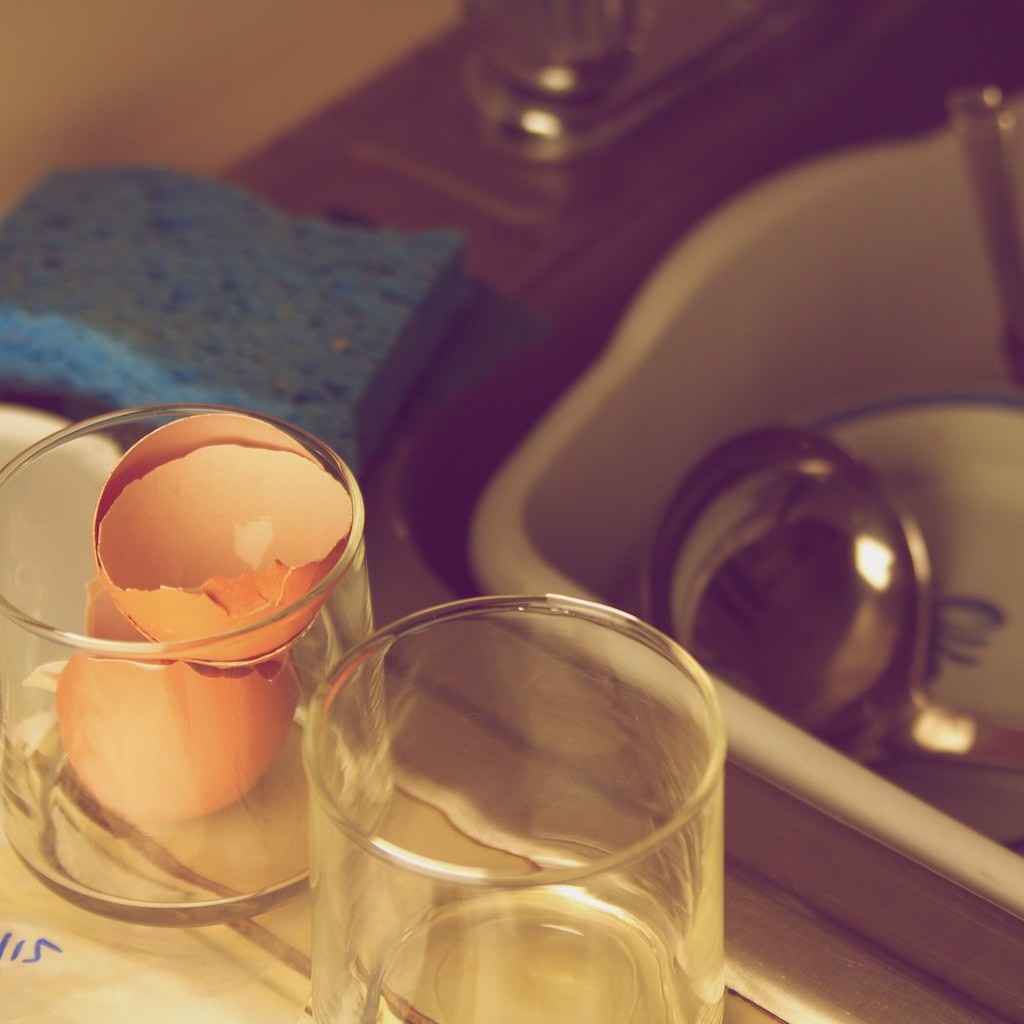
(735, 392), (1023, 854)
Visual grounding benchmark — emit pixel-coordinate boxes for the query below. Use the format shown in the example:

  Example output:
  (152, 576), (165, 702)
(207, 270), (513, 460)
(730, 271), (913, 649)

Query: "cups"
(1, 405), (394, 928)
(300, 590), (728, 1024)
